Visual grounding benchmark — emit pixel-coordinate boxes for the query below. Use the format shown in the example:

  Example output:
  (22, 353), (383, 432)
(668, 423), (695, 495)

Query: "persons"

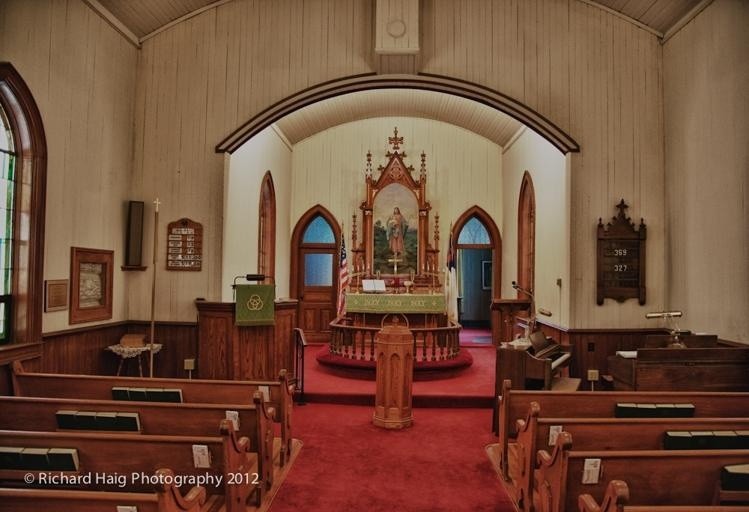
(386, 207), (409, 258)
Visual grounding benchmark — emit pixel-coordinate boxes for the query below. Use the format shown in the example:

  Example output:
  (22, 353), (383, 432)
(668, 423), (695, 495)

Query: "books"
(116, 504), (137, 512)
(616, 402), (695, 418)
(719, 460), (749, 506)
(192, 443), (210, 469)
(258, 384), (272, 402)
(112, 386), (183, 403)
(582, 458), (601, 484)
(362, 279), (387, 293)
(225, 410), (240, 431)
(614, 349), (639, 359)
(547, 424), (564, 447)
(55, 409), (142, 432)
(0, 445), (80, 487)
(663, 428), (749, 450)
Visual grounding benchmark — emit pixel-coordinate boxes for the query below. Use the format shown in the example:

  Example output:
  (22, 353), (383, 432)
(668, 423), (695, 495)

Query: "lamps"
(645, 311), (688, 348)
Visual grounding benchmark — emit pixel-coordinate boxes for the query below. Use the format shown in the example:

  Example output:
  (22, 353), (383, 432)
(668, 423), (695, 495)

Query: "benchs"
(0, 361), (302, 512)
(483, 378), (748, 512)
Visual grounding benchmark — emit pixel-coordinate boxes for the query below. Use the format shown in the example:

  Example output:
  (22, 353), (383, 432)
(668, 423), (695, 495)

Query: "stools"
(550, 378), (582, 393)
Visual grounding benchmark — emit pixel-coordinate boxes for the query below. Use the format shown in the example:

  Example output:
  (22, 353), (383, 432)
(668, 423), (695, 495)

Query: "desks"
(105, 344), (162, 377)
(345, 293), (446, 327)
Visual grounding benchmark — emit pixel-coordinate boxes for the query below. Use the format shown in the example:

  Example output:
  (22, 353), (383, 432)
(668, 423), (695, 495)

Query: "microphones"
(512, 280), (516, 285)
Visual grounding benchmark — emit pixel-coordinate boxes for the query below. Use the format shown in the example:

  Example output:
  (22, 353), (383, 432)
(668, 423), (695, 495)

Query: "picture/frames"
(45, 246), (115, 326)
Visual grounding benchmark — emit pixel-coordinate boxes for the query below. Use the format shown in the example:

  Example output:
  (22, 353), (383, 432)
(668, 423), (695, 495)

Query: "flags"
(444, 231), (459, 328)
(335, 231), (350, 327)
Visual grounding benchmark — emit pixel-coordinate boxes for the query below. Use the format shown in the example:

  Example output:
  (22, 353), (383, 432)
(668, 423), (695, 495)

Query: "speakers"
(125, 200), (144, 266)
